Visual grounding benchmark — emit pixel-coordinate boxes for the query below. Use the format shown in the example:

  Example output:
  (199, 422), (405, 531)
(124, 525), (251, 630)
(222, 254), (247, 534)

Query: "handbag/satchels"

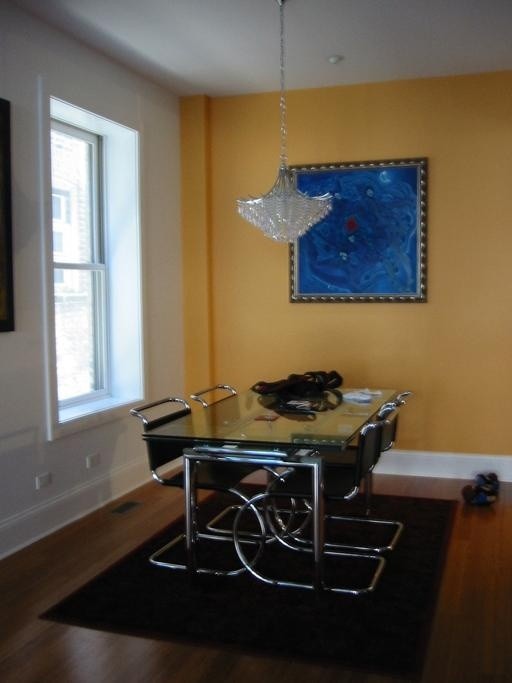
(250, 370), (343, 395)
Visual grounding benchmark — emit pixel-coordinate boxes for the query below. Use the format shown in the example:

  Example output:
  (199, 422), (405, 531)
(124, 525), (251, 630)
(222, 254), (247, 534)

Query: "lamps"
(233, 0), (333, 242)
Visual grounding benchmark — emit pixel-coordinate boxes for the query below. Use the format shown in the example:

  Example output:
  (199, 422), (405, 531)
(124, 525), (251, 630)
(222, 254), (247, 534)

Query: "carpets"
(38, 482), (460, 683)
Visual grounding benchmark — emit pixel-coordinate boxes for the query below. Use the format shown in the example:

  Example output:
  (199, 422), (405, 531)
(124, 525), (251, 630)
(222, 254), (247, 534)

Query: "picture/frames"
(288, 156), (429, 308)
